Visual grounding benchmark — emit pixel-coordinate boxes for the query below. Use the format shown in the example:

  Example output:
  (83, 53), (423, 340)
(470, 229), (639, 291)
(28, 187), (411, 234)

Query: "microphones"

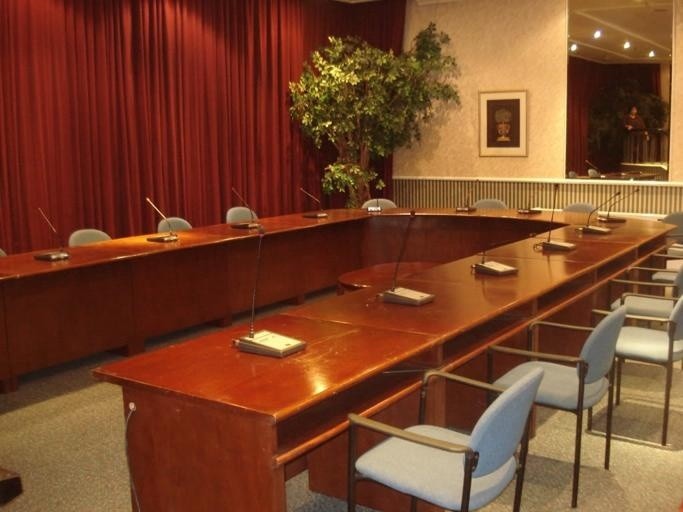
(518, 184), (539, 213)
(383, 210), (435, 307)
(229, 185), (260, 228)
(600, 187), (641, 223)
(144, 194), (177, 243)
(299, 185), (328, 216)
(34, 204), (71, 260)
(239, 225), (307, 357)
(475, 217), (521, 274)
(584, 191), (615, 233)
(584, 159), (605, 178)
(541, 181), (575, 251)
(457, 180), (480, 213)
(369, 179), (386, 211)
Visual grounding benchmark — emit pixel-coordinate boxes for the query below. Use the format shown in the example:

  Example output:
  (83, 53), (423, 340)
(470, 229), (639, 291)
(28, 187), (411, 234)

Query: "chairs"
(589, 169), (600, 178)
(568, 171), (576, 179)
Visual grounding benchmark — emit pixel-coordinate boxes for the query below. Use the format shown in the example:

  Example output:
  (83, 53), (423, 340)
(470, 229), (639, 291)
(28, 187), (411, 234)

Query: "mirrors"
(565, 0), (675, 181)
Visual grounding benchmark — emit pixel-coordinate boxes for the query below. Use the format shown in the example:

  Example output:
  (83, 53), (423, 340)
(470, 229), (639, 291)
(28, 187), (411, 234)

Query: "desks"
(600, 171), (657, 180)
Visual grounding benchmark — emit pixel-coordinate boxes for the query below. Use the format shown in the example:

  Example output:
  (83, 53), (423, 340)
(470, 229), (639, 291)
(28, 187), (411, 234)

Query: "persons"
(620, 105), (650, 130)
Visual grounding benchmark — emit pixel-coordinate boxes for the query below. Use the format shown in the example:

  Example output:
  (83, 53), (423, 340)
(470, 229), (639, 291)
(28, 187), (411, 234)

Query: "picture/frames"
(479, 91), (527, 158)
